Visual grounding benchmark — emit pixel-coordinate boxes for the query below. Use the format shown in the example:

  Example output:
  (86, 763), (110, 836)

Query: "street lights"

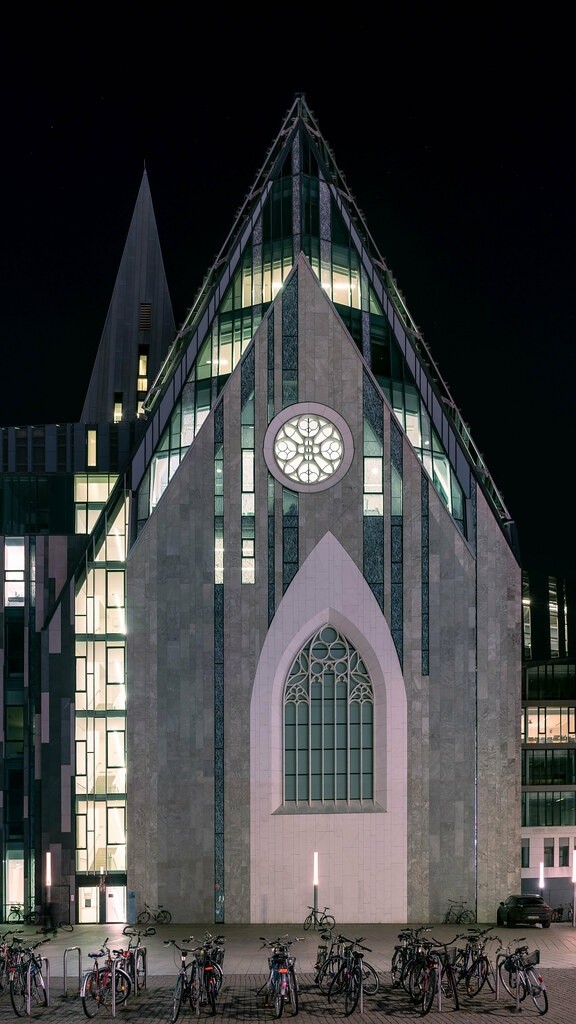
(571, 850), (576, 930)
(539, 862), (545, 896)
(44, 852), (52, 934)
(313, 852), (319, 930)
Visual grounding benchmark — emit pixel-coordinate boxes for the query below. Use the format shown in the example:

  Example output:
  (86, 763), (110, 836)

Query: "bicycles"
(303, 905), (336, 932)
(136, 903), (171, 925)
(162, 939), (211, 1024)
(182, 934), (218, 1017)
(446, 929), (503, 997)
(259, 933), (289, 1018)
(444, 899), (475, 925)
(56, 920), (74, 932)
(327, 937), (366, 1016)
(337, 933), (381, 995)
(8, 904), (40, 926)
(428, 933), (460, 1010)
(389, 926), (438, 1014)
(314, 934), (352, 996)
(466, 927), (494, 991)
(0, 930), (47, 1018)
(495, 938), (549, 1015)
(80, 938), (132, 1019)
(259, 936), (303, 1016)
(197, 931), (229, 971)
(99, 924), (156, 1006)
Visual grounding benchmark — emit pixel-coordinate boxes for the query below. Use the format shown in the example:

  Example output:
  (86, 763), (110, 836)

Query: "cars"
(496, 894), (553, 928)
